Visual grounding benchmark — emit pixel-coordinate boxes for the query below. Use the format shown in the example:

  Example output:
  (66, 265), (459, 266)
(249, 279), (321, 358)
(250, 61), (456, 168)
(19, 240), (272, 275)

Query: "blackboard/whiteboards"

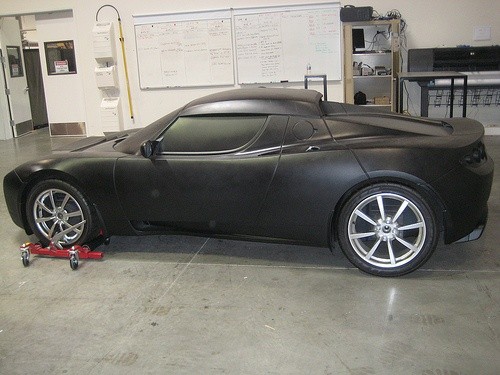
(134, 17), (236, 91)
(233, 6), (343, 86)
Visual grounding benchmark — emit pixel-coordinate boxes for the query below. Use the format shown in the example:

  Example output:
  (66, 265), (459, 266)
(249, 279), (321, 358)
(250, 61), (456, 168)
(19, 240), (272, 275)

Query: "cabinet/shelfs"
(342, 19), (401, 114)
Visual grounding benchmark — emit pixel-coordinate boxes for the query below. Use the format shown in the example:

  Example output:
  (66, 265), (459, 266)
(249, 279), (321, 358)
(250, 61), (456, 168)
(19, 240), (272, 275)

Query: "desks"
(393, 71), (467, 119)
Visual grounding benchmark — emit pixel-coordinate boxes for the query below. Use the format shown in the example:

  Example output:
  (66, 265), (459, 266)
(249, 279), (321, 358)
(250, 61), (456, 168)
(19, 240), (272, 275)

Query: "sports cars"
(3, 86), (495, 279)
